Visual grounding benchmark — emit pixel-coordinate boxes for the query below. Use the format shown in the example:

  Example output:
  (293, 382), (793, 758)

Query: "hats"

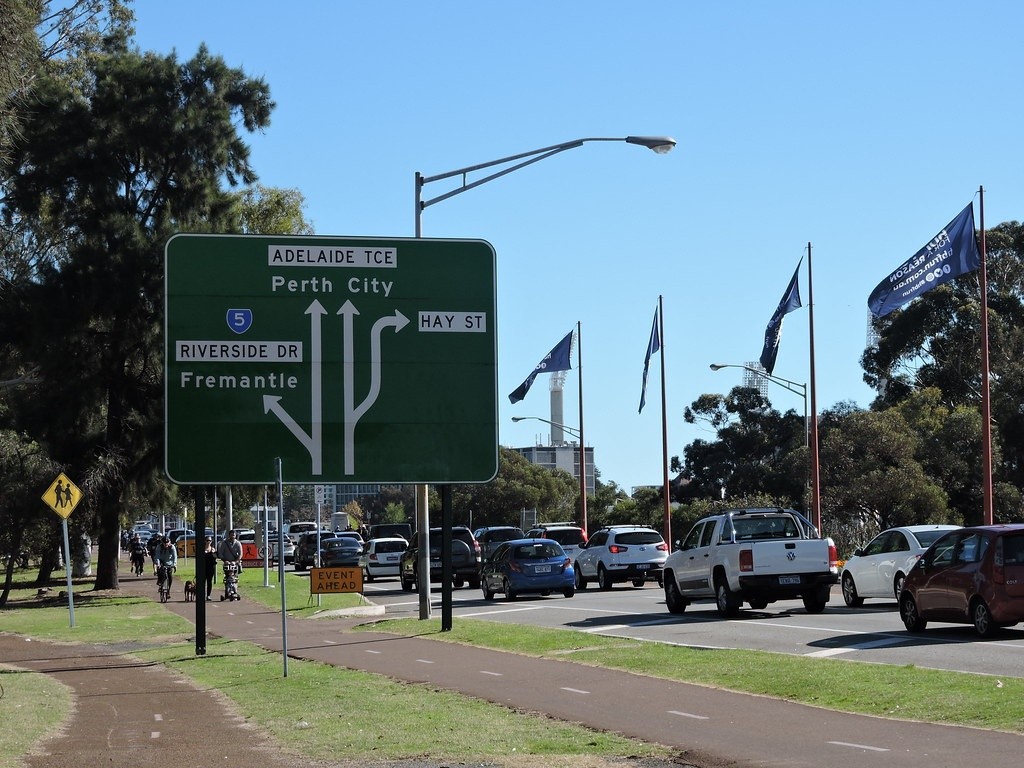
(162, 535), (170, 543)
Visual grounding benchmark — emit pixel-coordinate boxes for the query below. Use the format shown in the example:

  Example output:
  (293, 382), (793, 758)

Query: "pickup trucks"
(662, 506), (841, 618)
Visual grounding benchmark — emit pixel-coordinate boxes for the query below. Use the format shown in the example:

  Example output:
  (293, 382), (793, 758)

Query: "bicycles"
(157, 565), (176, 603)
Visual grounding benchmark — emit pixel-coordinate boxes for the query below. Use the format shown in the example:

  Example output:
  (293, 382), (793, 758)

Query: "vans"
(472, 526), (526, 565)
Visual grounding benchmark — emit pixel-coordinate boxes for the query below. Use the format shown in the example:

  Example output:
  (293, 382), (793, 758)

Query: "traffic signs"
(162, 235), (499, 487)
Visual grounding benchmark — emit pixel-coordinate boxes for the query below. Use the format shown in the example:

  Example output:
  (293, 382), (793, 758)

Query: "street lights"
(511, 417), (587, 535)
(412, 133), (678, 623)
(709, 363), (811, 537)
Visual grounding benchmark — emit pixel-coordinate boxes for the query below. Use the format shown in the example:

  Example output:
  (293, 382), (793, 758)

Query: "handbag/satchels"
(237, 563), (243, 574)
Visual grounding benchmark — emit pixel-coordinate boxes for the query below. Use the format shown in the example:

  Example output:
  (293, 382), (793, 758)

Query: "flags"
(866, 203), (980, 316)
(638, 306), (660, 414)
(508, 329), (574, 403)
(760, 255), (805, 377)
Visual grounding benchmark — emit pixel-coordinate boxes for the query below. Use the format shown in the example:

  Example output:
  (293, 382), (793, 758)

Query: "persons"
(121, 527), (147, 577)
(273, 528), (277, 533)
(323, 526), (326, 530)
(219, 530), (243, 596)
(205, 537), (216, 601)
(335, 524), (367, 538)
(146, 533), (178, 598)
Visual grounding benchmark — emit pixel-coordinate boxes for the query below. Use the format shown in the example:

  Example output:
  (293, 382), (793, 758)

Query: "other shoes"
(137, 574), (140, 577)
(156, 580), (159, 585)
(130, 567), (134, 573)
(166, 593), (171, 599)
(207, 596), (212, 601)
(154, 572), (157, 576)
(140, 572), (143, 575)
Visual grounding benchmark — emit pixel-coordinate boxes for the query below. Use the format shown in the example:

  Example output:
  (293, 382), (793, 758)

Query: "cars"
(480, 538), (576, 601)
(131, 519), (417, 584)
(898, 523), (1024, 638)
(840, 525), (965, 608)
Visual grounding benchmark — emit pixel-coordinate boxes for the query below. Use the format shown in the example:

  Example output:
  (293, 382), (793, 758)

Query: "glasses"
(206, 539), (213, 542)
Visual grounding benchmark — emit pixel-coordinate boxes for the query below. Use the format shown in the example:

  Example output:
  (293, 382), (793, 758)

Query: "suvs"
(573, 525), (670, 591)
(522, 520), (590, 571)
(398, 526), (483, 593)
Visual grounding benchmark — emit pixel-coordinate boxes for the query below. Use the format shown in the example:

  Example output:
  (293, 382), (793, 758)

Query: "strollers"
(219, 562), (242, 602)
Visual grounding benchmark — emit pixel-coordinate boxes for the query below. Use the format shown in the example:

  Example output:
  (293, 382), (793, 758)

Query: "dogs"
(184, 580), (197, 602)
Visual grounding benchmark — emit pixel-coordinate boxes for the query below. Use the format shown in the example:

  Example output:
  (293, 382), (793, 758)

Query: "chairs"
(755, 525), (772, 534)
